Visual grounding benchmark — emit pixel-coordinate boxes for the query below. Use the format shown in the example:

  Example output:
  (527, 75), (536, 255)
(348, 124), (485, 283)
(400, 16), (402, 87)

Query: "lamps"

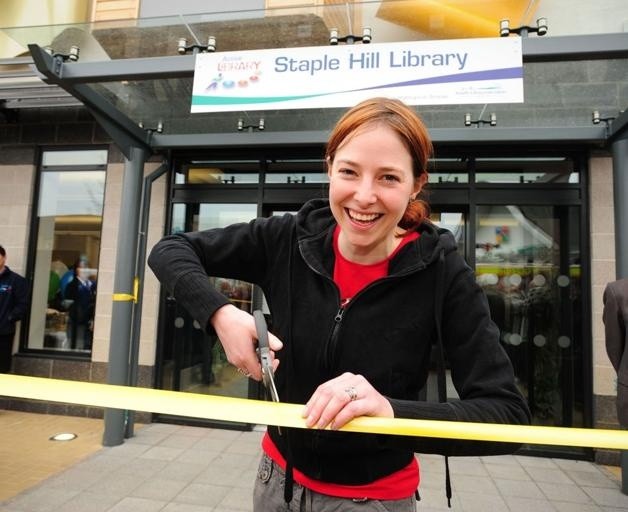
(592, 109), (625, 125)
(500, 17), (548, 37)
(237, 118), (265, 132)
(43, 46), (79, 62)
(329, 26), (372, 45)
(464, 112), (496, 128)
(138, 120), (164, 135)
(177, 36), (217, 56)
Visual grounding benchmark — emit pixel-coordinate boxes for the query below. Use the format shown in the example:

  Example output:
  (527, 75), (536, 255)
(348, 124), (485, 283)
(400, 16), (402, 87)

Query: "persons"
(2, 247), (29, 375)
(516, 258), (547, 298)
(146, 96), (534, 512)
(601, 278), (628, 493)
(60, 258), (97, 353)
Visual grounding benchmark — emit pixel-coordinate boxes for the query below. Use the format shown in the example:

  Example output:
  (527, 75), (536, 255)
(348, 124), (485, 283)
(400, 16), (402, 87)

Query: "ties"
(85, 280), (90, 292)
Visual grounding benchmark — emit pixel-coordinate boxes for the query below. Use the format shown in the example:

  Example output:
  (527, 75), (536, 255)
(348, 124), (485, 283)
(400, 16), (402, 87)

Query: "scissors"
(252, 309), (282, 436)
